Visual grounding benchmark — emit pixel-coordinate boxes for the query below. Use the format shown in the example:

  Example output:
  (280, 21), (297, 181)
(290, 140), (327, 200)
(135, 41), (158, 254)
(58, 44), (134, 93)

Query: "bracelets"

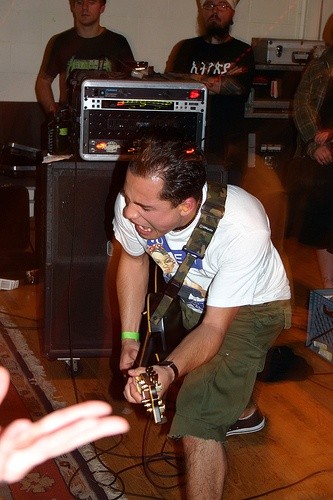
(121, 331), (139, 340)
(158, 361), (179, 382)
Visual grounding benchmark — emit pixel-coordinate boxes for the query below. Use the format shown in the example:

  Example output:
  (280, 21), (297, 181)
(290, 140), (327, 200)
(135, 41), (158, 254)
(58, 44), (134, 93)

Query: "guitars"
(121, 262), (173, 424)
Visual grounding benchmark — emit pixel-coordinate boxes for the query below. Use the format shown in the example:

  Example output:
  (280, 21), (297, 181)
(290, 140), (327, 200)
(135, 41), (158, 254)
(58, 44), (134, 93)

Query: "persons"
(0, 365), (130, 484)
(34, 0), (136, 114)
(164, 0), (252, 150)
(112, 135), (291, 500)
(292, 13), (333, 289)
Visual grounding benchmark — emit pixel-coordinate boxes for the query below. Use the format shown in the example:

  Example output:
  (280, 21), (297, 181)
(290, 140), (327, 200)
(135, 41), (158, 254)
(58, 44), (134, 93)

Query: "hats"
(199, 0), (240, 11)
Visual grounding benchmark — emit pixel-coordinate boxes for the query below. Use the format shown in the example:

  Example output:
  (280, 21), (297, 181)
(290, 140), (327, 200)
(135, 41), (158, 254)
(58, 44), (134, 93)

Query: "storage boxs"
(245, 37), (325, 170)
(306, 288), (333, 362)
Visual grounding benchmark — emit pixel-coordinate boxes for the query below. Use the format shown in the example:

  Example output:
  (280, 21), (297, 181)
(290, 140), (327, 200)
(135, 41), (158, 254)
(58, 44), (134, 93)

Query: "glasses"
(202, 2), (231, 11)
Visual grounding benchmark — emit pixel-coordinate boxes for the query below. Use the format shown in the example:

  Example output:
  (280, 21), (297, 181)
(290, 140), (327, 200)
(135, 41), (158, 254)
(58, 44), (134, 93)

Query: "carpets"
(0, 307), (126, 500)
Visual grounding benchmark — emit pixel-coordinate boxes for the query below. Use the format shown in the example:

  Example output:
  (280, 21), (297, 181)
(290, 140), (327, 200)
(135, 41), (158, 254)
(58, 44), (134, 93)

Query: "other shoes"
(224, 406), (266, 435)
